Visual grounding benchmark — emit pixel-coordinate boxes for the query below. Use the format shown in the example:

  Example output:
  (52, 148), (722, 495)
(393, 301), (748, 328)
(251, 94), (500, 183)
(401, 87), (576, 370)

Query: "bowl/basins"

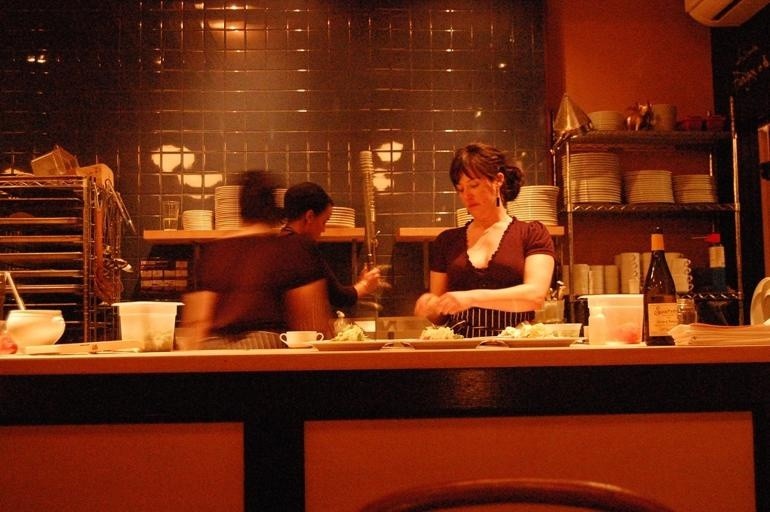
(6, 310), (66, 354)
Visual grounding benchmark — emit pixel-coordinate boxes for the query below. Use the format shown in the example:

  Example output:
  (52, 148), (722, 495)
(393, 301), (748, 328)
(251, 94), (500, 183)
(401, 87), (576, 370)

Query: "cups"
(575, 251), (694, 296)
(279, 330), (324, 349)
(535, 299), (564, 325)
(162, 201), (180, 231)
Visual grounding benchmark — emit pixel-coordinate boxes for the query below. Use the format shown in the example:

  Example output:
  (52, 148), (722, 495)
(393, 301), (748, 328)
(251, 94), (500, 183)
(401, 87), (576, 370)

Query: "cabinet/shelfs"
(550, 130), (745, 336)
(0, 174), (123, 342)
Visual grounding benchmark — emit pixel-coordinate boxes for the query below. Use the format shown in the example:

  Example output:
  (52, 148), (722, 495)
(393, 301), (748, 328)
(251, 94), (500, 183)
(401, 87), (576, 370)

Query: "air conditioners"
(684, 0), (770, 27)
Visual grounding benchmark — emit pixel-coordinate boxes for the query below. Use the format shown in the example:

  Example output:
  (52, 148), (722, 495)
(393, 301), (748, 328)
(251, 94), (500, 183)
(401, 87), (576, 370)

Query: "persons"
(181, 171), (383, 339)
(413, 143), (556, 337)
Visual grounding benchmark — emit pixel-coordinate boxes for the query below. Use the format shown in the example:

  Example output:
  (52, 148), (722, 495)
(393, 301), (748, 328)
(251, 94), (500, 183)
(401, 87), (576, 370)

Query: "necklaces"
(478, 225), (490, 237)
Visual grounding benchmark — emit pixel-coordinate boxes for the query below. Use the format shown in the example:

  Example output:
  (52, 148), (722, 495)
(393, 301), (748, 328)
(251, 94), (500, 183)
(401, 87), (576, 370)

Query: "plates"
(650, 103), (676, 133)
(325, 207), (356, 228)
(273, 189), (288, 207)
(311, 341), (386, 351)
(561, 153), (719, 204)
(183, 185), (244, 230)
(502, 337), (580, 347)
(412, 339), (481, 350)
(589, 111), (624, 130)
(457, 185), (560, 227)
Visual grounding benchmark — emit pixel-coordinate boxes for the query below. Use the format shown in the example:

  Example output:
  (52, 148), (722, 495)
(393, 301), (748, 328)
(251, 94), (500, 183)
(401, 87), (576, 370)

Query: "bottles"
(644, 233), (677, 346)
(708, 233), (726, 290)
(677, 298), (697, 325)
